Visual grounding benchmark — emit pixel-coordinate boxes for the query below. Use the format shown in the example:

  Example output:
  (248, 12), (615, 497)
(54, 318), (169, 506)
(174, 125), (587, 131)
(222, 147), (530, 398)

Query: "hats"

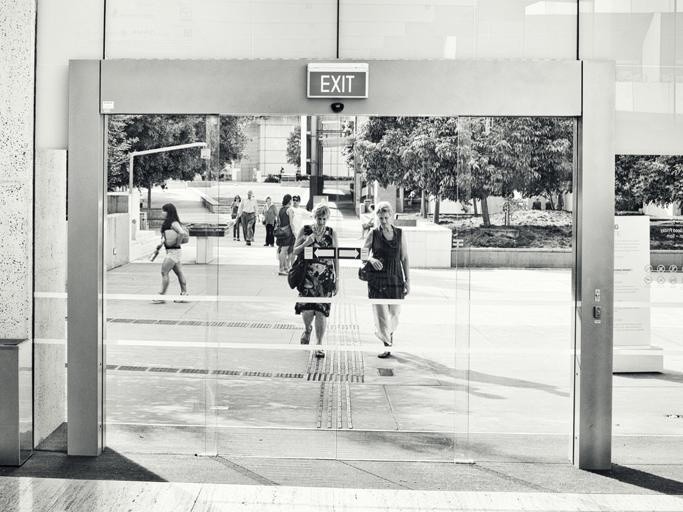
(292, 195), (301, 200)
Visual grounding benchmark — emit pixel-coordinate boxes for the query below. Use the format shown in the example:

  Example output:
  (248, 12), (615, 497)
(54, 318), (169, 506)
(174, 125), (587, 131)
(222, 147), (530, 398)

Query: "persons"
(230, 190), (301, 276)
(292, 203), (340, 357)
(150, 202), (189, 304)
(361, 204), (375, 240)
(361, 201), (410, 359)
(545, 198), (556, 210)
(532, 197), (541, 210)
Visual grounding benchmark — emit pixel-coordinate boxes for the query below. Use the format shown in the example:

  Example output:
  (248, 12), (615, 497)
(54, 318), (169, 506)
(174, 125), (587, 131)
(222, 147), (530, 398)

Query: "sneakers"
(151, 295), (166, 303)
(378, 349), (390, 358)
(174, 293), (190, 303)
(315, 346), (325, 359)
(300, 326), (313, 344)
(278, 267), (291, 276)
(231, 238), (274, 247)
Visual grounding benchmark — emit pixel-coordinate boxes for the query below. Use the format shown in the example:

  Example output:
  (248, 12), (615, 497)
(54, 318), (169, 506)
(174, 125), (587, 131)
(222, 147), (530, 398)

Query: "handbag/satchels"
(287, 252), (309, 289)
(357, 258), (378, 282)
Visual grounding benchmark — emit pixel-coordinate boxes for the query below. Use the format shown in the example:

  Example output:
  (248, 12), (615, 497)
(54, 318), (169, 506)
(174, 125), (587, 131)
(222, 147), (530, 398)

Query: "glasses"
(293, 198), (298, 202)
